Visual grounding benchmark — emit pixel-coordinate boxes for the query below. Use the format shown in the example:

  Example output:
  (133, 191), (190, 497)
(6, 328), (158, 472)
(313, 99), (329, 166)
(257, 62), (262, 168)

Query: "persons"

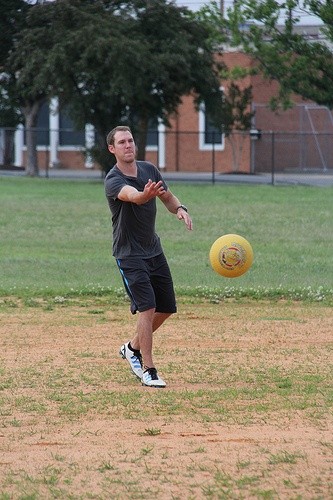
(104, 126), (192, 388)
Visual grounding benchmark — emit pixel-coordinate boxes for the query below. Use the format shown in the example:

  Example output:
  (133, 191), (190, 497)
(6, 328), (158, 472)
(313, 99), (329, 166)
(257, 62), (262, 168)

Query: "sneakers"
(119, 341), (143, 379)
(141, 365), (166, 386)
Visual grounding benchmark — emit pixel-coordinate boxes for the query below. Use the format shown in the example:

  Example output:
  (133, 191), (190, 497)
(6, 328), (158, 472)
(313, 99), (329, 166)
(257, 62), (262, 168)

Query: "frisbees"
(208, 233), (253, 278)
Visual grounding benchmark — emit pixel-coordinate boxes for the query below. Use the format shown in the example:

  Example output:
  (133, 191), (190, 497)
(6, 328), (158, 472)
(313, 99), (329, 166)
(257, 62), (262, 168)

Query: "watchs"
(177, 205), (188, 212)
